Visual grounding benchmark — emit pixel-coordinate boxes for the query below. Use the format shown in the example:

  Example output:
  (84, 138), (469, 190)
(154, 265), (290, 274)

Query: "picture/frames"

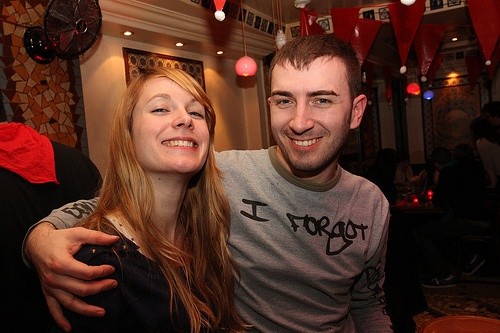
(122, 47), (206, 94)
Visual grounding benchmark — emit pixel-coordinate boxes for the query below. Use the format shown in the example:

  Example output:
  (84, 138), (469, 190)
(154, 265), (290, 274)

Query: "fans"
(24, 0), (102, 64)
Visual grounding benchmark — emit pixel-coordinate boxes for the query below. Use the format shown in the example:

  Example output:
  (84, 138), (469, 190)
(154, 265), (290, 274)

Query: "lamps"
(234, 0), (257, 77)
(275, 0), (286, 50)
(407, 59), (434, 100)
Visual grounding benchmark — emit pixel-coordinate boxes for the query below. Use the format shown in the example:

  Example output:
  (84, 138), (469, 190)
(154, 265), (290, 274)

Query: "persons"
(19, 33), (500, 333)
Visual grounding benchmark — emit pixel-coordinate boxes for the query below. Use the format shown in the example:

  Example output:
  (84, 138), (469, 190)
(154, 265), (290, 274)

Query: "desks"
(392, 207), (446, 287)
(418, 315), (500, 333)
